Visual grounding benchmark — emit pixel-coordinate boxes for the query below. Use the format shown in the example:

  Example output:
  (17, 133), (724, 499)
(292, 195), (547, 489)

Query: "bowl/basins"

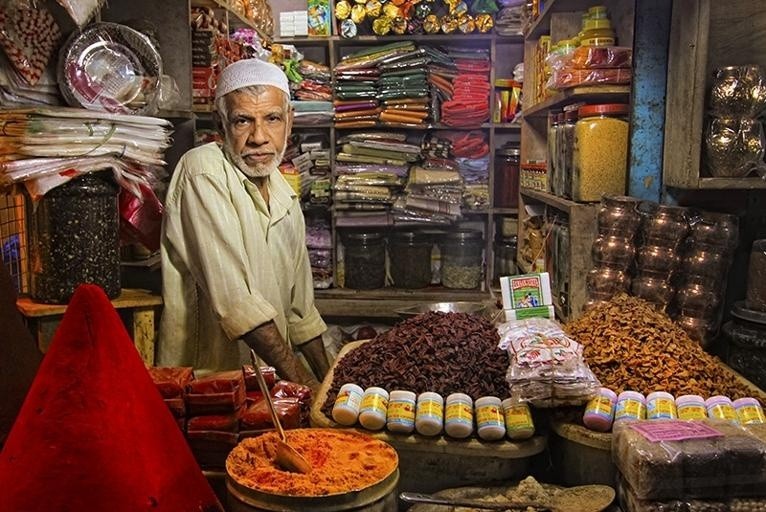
(57, 21), (162, 117)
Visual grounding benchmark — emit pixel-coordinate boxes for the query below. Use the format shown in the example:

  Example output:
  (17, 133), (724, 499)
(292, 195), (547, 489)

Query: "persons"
(154, 59), (332, 404)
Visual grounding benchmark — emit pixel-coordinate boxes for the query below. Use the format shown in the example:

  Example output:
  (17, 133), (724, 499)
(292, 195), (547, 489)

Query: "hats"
(214, 58), (291, 98)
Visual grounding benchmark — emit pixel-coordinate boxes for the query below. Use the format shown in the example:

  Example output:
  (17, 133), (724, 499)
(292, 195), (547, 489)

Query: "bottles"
(546, 103), (630, 204)
(390, 227), (433, 289)
(29, 166), (121, 306)
(493, 234), (519, 289)
(331, 383), (536, 442)
(345, 228), (387, 289)
(442, 230), (484, 289)
(496, 149), (518, 208)
(583, 388), (762, 432)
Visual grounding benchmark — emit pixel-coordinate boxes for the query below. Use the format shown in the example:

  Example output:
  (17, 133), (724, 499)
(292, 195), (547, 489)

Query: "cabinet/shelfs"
(516, 0), (634, 324)
(189, 0), (272, 140)
(272, 35), (525, 319)
